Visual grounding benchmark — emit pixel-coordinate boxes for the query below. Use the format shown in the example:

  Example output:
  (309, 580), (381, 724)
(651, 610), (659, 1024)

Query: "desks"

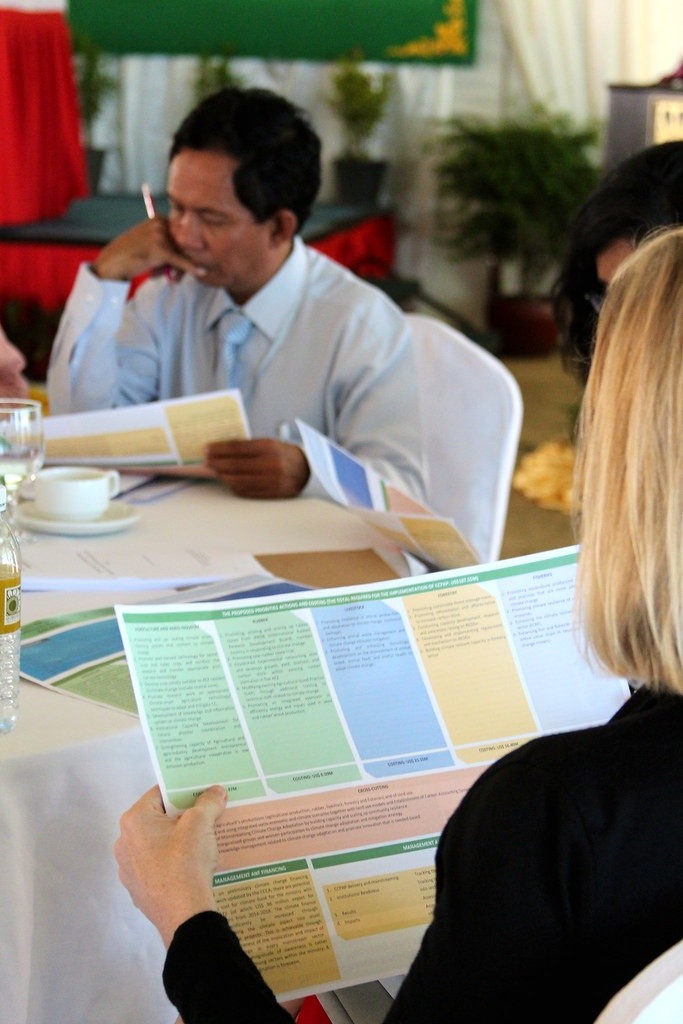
(2, 480), (412, 1023)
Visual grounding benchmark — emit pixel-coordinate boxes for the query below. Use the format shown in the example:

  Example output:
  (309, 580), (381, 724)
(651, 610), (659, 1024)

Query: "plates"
(12, 500), (139, 534)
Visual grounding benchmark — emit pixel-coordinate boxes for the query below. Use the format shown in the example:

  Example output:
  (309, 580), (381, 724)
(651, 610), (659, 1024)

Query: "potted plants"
(320, 52), (387, 207)
(73, 47), (117, 194)
(418, 108), (603, 359)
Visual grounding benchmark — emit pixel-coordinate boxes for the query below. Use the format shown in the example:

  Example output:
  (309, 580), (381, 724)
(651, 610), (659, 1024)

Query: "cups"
(0, 397), (45, 499)
(34, 465), (121, 523)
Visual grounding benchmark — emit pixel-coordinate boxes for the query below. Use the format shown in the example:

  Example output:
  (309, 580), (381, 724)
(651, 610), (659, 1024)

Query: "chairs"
(404, 313), (524, 563)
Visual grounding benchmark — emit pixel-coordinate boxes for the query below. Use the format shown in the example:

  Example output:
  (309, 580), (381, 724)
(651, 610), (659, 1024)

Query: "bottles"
(0, 485), (22, 735)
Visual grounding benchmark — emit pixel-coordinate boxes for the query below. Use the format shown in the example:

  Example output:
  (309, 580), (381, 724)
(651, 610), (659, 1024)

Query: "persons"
(47, 85), (431, 507)
(115, 228), (683, 1023)
(549, 138), (683, 440)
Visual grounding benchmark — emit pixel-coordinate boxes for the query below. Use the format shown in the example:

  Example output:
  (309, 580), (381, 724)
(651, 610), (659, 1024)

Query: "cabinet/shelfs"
(605, 85), (683, 181)
